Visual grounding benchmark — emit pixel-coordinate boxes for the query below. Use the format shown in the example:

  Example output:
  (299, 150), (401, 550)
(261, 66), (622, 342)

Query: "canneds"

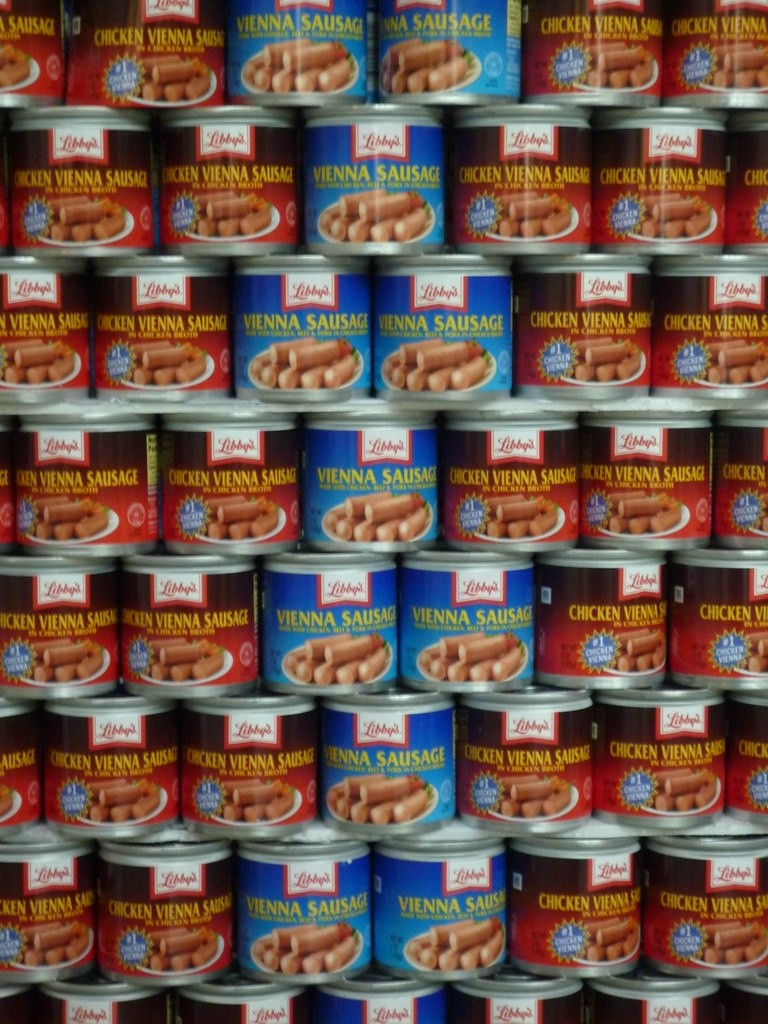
(0, 0), (768, 1024)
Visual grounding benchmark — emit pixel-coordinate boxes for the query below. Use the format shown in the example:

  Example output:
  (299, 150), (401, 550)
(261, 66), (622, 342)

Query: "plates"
(2, 43), (767, 977)
